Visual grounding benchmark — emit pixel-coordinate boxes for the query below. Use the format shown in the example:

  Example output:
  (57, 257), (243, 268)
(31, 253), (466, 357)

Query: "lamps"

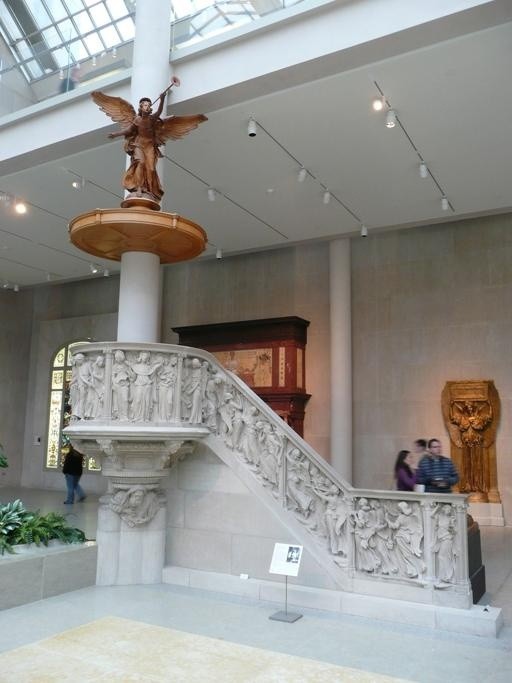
(322, 190), (331, 203)
(419, 161), (427, 180)
(441, 195), (448, 211)
(1, 163), (110, 294)
(207, 187), (216, 202)
(215, 250), (222, 260)
(247, 120), (257, 138)
(360, 223), (369, 237)
(385, 110), (397, 127)
(298, 168), (309, 183)
(373, 94), (386, 112)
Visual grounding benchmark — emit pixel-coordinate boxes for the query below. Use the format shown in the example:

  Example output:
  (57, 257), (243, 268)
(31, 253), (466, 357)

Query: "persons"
(349, 498), (455, 586)
(448, 400), (493, 492)
(68, 351), (217, 425)
(62, 443), (87, 505)
(395, 438), (460, 493)
(91, 89), (208, 196)
(214, 385), (346, 557)
